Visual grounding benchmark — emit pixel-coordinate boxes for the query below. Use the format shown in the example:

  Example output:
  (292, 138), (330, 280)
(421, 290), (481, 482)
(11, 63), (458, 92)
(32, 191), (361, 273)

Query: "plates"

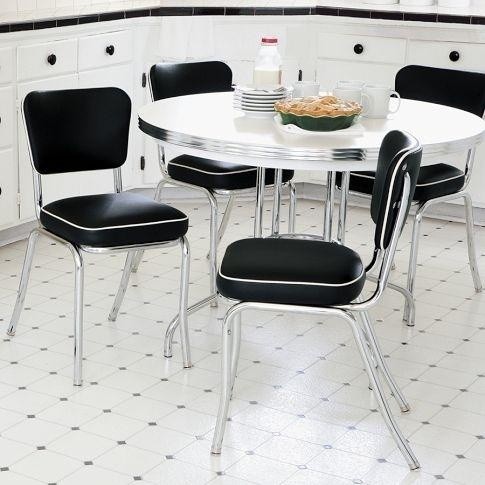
(231, 85), (293, 120)
(274, 97), (363, 131)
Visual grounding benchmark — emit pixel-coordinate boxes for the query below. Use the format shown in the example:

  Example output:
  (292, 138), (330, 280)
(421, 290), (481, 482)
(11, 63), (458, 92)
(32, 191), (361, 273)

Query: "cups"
(332, 79), (370, 118)
(293, 81), (321, 98)
(362, 83), (401, 119)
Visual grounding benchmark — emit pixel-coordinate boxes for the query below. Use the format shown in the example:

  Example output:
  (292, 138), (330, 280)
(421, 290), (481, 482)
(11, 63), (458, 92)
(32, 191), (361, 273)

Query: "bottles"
(253, 35), (284, 87)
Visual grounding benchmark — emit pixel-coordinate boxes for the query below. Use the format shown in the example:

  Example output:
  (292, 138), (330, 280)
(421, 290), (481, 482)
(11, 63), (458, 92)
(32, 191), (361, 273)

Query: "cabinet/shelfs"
(1, 17), (145, 248)
(316, 15), (485, 226)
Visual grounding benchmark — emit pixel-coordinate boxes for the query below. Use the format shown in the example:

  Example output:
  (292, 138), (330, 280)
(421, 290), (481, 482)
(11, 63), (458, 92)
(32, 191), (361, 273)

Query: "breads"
(275, 96), (361, 117)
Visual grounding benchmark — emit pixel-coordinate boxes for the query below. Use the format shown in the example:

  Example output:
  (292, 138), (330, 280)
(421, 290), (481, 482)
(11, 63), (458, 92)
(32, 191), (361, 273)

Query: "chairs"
(6, 84), (194, 388)
(325, 61), (484, 326)
(204, 127), (425, 472)
(132, 58), (300, 314)
